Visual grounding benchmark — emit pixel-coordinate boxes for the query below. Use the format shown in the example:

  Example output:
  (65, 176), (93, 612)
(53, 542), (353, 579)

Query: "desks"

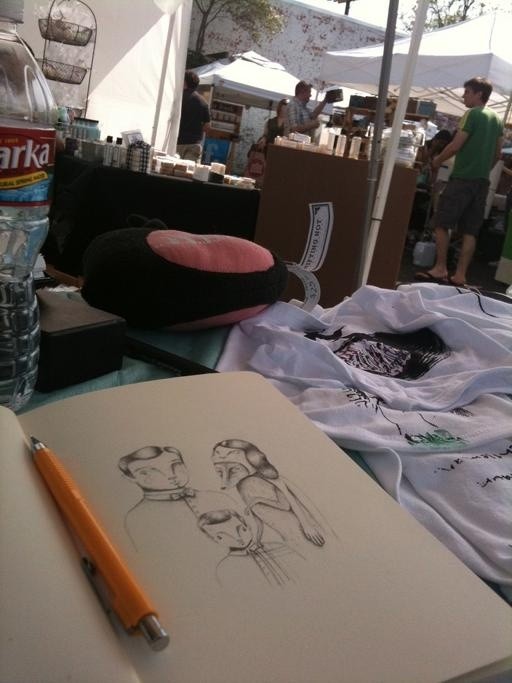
(47, 153), (261, 297)
(253, 144), (419, 312)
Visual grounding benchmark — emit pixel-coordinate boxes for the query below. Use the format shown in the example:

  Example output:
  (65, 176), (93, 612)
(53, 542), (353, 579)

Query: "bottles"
(56, 116), (127, 171)
(347, 136), (362, 160)
(334, 134), (346, 158)
(0, 0), (58, 412)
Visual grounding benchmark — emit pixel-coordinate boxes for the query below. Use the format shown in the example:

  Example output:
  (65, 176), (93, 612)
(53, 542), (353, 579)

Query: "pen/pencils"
(29, 433), (170, 653)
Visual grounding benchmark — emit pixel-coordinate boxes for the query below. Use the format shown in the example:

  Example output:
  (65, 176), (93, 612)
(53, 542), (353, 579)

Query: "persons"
(176, 70), (211, 162)
(401, 116), (512, 264)
(411, 75), (505, 288)
(245, 81), (396, 189)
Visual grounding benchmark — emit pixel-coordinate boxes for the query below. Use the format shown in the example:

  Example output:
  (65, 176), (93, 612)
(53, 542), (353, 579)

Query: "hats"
(296, 81), (312, 92)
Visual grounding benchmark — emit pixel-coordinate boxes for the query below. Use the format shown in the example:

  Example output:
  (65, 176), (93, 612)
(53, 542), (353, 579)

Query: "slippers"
(438, 274), (465, 286)
(414, 271), (448, 284)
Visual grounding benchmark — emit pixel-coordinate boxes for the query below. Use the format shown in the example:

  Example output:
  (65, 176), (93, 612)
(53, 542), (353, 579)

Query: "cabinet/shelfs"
(38, 0), (98, 120)
